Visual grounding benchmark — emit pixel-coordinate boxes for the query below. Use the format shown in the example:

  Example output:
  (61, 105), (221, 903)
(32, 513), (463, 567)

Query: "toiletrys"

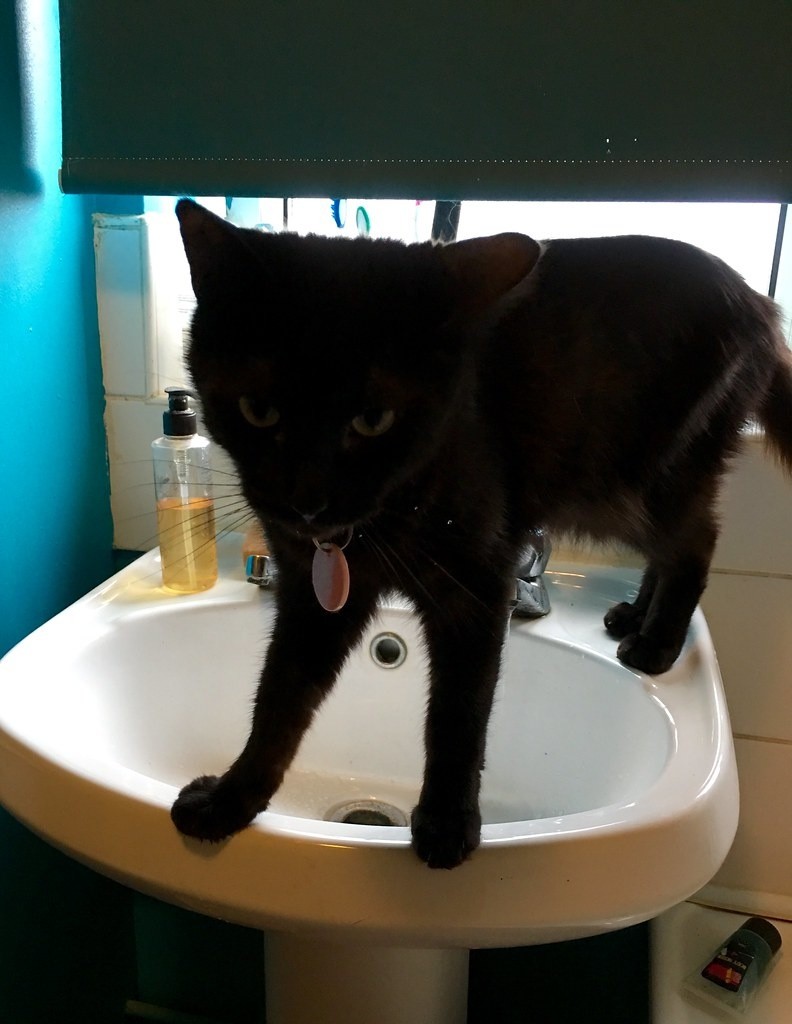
(150, 386), (222, 595)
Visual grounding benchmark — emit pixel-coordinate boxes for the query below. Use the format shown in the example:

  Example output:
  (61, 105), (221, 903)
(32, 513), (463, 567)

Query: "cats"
(172, 200), (792, 869)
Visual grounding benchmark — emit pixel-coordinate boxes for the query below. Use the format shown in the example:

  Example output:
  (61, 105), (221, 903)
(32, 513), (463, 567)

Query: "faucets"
(510, 529), (553, 620)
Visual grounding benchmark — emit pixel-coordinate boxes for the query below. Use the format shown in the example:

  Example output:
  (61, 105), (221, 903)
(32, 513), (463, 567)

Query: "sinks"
(0, 534), (743, 951)
